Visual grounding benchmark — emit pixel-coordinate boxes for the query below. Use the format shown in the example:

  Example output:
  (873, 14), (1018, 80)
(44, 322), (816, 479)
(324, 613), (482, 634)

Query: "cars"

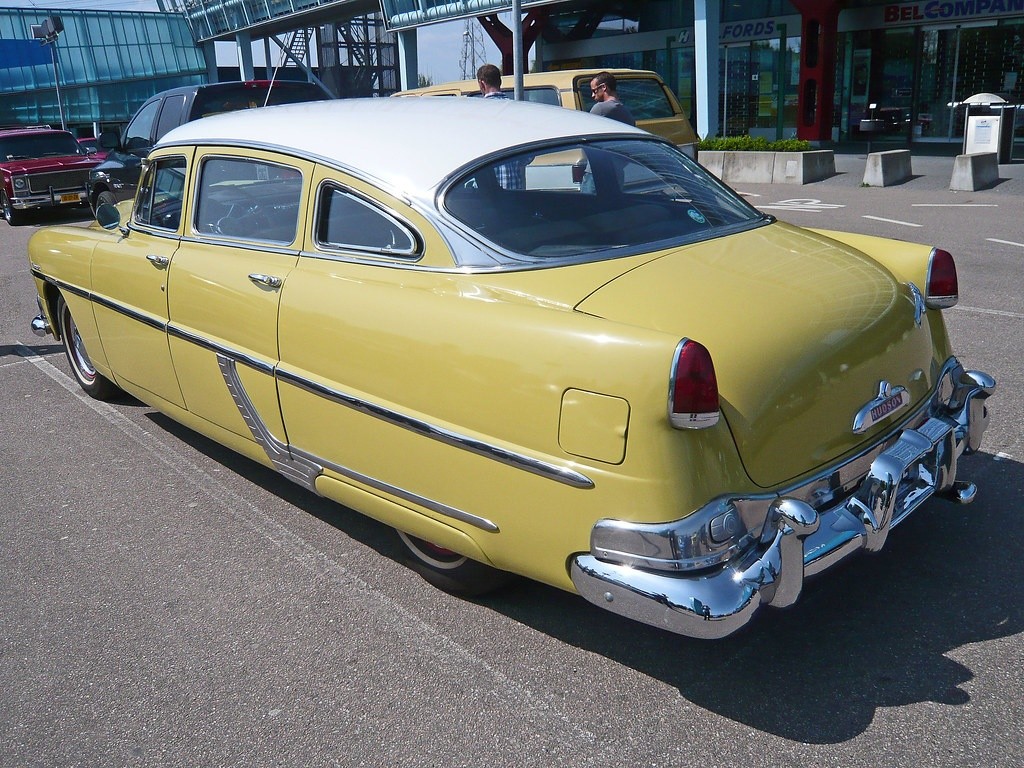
(26, 94), (998, 651)
(858, 107), (933, 134)
(76, 134), (108, 163)
(0, 124), (104, 221)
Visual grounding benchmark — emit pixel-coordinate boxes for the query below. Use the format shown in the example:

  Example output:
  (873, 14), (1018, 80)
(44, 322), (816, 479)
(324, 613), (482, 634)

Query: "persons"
(470, 63), (535, 192)
(578, 71), (637, 196)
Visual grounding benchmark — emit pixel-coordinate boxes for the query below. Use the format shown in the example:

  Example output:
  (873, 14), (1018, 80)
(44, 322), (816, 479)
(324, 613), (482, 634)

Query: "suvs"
(87, 77), (339, 221)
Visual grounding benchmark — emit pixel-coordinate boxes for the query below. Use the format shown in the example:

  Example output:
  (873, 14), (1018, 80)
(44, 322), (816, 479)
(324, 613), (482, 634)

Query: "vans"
(386, 67), (700, 166)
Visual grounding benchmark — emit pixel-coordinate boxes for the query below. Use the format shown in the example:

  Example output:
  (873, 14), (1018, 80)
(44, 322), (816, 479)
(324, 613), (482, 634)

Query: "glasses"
(591, 83), (605, 94)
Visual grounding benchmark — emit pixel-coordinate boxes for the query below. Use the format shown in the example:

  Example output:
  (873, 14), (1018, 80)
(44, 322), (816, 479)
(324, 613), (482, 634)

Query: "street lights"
(30, 16), (70, 130)
